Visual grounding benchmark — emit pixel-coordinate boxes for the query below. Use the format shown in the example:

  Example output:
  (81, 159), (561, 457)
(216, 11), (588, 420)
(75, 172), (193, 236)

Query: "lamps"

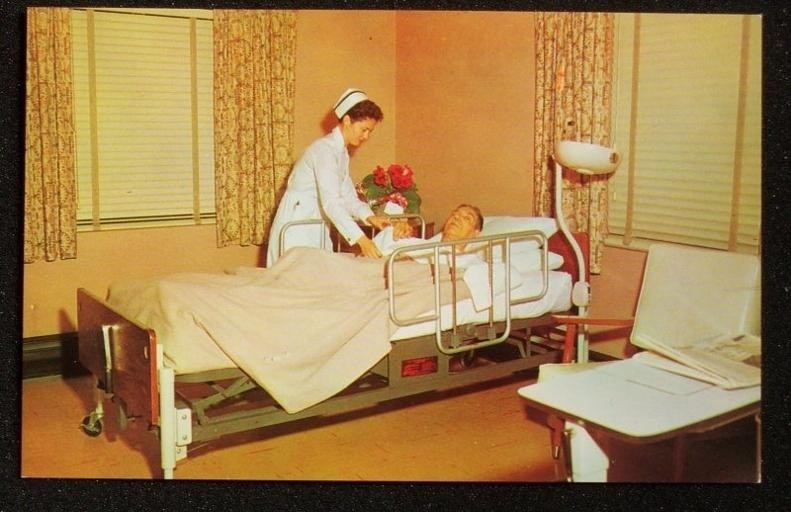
(546, 137), (623, 436)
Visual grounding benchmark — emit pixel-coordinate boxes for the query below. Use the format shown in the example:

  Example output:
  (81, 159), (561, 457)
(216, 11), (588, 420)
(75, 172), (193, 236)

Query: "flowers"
(352, 159), (422, 210)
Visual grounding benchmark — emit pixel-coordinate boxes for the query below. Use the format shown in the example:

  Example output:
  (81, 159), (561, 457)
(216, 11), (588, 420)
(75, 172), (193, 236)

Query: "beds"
(71, 208), (599, 481)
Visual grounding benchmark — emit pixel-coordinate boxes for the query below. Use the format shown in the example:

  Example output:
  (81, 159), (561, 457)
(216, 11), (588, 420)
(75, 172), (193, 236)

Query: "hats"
(332, 87), (368, 121)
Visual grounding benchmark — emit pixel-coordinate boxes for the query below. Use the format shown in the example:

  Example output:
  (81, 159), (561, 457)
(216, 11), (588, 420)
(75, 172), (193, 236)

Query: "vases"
(377, 199), (405, 217)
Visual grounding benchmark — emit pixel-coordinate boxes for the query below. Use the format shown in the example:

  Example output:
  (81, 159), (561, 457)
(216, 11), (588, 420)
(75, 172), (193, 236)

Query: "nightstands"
(325, 219), (437, 259)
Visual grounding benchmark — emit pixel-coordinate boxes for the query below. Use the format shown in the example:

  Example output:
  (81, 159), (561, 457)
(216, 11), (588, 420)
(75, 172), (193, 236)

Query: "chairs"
(533, 238), (761, 482)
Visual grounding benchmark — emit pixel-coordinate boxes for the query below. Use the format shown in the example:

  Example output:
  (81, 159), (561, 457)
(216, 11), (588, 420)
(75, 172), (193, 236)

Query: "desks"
(513, 344), (764, 484)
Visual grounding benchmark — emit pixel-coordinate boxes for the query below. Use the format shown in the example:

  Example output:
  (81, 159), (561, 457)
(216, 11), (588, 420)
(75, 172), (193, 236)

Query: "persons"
(392, 202), (486, 270)
(266, 87), (392, 268)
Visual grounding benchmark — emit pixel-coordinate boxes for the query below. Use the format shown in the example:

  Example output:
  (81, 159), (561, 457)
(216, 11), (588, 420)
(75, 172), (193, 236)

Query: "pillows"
(426, 215), (561, 268)
(505, 244), (568, 278)
